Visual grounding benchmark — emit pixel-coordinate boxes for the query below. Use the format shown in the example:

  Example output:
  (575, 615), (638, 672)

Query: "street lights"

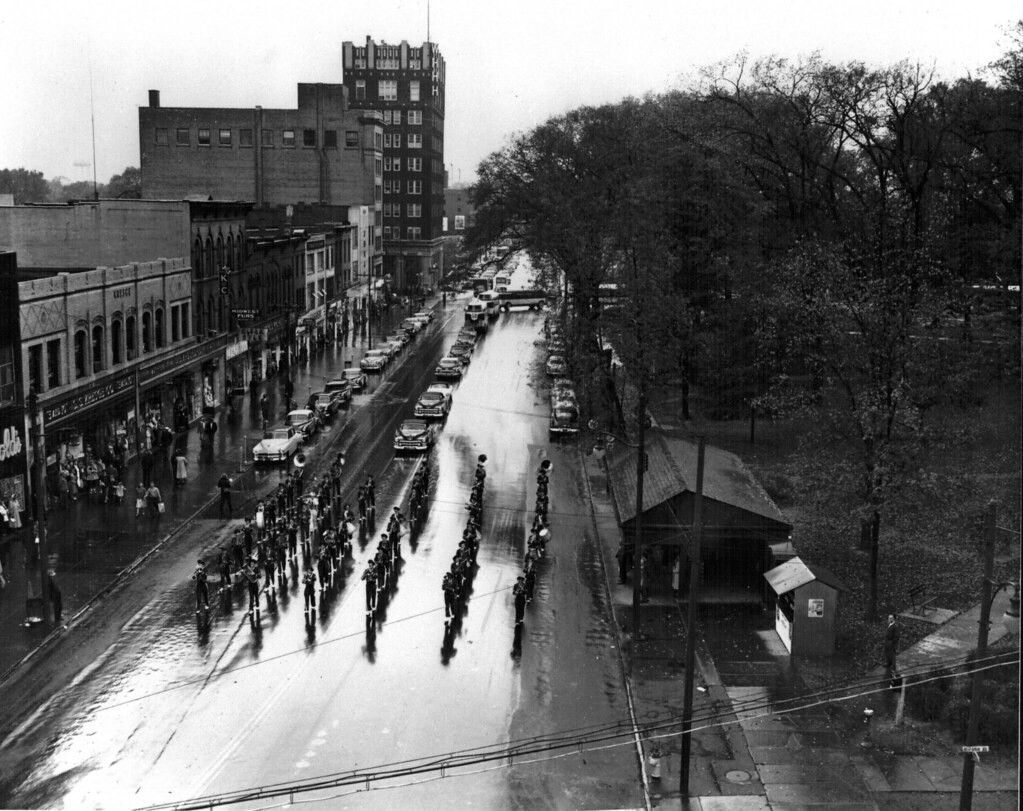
(593, 394), (647, 640)
(958, 499), (1020, 811)
(26, 386), (80, 621)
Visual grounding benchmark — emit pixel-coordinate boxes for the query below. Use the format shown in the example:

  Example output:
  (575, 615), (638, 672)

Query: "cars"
(413, 392), (449, 419)
(393, 419), (434, 451)
(427, 326), (477, 404)
(546, 313), (582, 435)
(252, 309), (436, 464)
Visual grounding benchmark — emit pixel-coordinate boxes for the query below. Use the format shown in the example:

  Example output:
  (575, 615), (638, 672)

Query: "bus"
(463, 289), (547, 333)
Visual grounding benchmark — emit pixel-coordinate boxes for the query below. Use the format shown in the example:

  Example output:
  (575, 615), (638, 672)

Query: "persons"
(615, 542), (628, 585)
(48, 568), (65, 621)
(57, 356), (294, 519)
(193, 450), (550, 648)
(0, 494), (22, 535)
(882, 614), (899, 670)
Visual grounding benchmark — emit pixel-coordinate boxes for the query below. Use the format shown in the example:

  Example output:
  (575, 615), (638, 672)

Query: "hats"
(221, 473), (227, 478)
(195, 463), (374, 576)
(365, 459), (429, 566)
(514, 468), (548, 583)
(440, 452), (487, 590)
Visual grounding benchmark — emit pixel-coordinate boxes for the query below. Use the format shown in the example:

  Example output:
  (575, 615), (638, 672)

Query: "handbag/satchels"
(158, 502), (165, 513)
